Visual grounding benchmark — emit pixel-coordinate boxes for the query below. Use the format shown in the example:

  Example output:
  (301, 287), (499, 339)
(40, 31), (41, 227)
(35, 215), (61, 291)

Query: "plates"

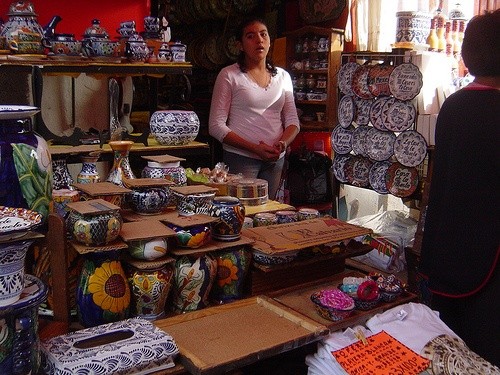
(330, 61), (428, 197)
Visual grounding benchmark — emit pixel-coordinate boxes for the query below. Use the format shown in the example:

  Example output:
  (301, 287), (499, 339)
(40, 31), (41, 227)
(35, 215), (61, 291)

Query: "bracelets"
(279, 141), (286, 152)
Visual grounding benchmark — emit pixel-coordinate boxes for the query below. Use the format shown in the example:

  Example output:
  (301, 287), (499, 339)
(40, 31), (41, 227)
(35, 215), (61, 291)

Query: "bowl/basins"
(337, 283), (383, 311)
(369, 275), (398, 303)
(311, 287), (356, 322)
(0, 0), (328, 375)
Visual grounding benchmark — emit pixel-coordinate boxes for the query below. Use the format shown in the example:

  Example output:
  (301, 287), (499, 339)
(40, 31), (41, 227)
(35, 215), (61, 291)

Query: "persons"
(208, 15), (301, 203)
(418, 7), (500, 368)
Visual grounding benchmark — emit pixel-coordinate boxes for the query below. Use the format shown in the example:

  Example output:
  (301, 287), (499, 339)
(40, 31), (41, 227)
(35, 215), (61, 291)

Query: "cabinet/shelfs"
(0, 192), (415, 375)
(285, 28), (345, 130)
(0, 50), (210, 155)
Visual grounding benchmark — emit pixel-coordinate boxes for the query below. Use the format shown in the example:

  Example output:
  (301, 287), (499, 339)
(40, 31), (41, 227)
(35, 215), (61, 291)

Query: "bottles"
(0, 104), (53, 235)
(427, 15), (465, 57)
